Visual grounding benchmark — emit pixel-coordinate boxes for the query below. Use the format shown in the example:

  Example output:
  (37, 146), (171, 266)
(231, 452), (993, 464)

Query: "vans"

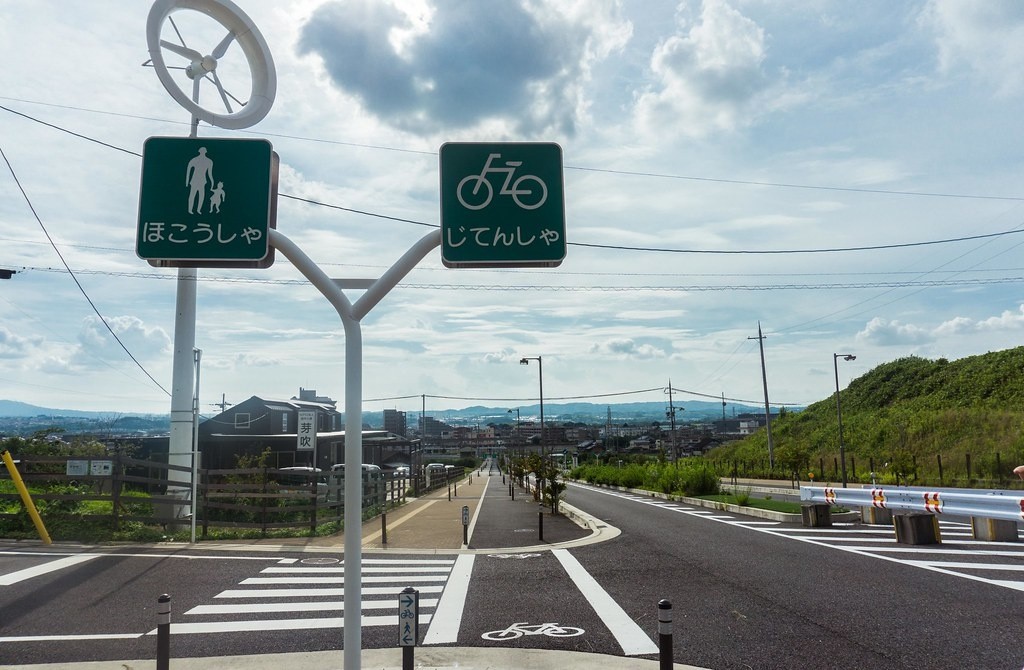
(277, 467), (330, 504)
(327, 464), (388, 508)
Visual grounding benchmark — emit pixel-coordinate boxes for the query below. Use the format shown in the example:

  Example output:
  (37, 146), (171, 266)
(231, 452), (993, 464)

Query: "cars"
(391, 466), (411, 479)
(426, 463), (456, 469)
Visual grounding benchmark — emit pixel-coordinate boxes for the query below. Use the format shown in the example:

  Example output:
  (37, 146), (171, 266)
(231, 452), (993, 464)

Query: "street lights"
(519, 356), (546, 503)
(832, 352), (855, 487)
(507, 409), (521, 491)
